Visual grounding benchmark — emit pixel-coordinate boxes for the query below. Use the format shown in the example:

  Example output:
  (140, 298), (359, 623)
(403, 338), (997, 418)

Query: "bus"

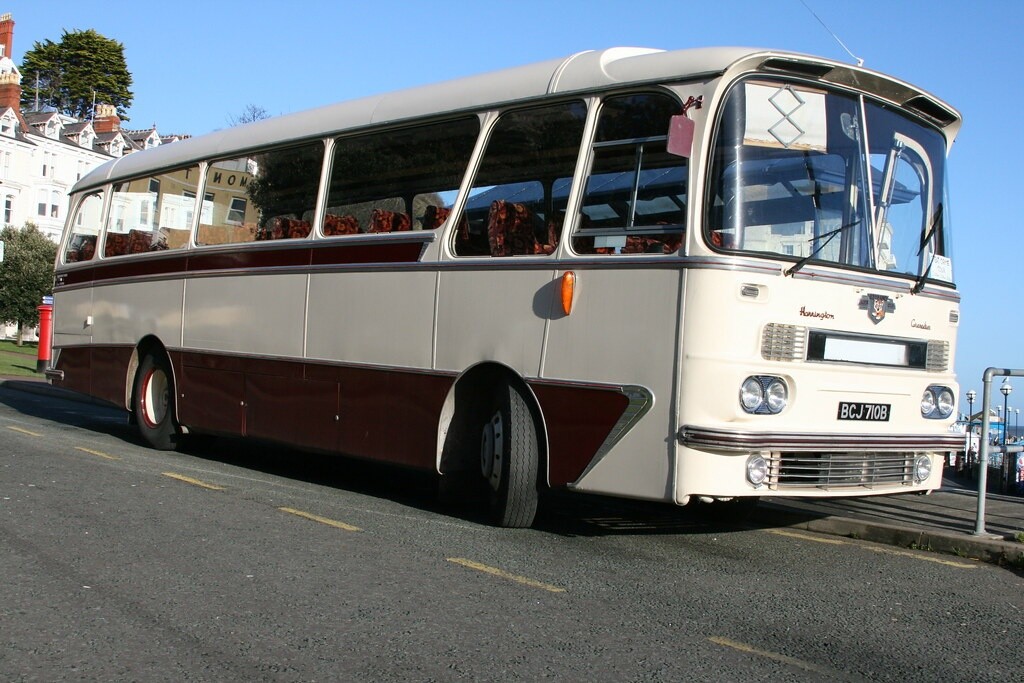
(43, 45), (967, 530)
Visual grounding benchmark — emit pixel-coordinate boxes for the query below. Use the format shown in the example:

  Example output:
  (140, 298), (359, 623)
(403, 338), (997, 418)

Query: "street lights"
(1015, 408), (1019, 443)
(965, 390), (976, 480)
(1007, 406), (1012, 441)
(996, 404), (1002, 443)
(1000, 383), (1012, 484)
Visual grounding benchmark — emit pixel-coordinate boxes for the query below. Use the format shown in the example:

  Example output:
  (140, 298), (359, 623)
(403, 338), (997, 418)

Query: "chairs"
(67, 231), (174, 263)
(258, 200), (725, 257)
